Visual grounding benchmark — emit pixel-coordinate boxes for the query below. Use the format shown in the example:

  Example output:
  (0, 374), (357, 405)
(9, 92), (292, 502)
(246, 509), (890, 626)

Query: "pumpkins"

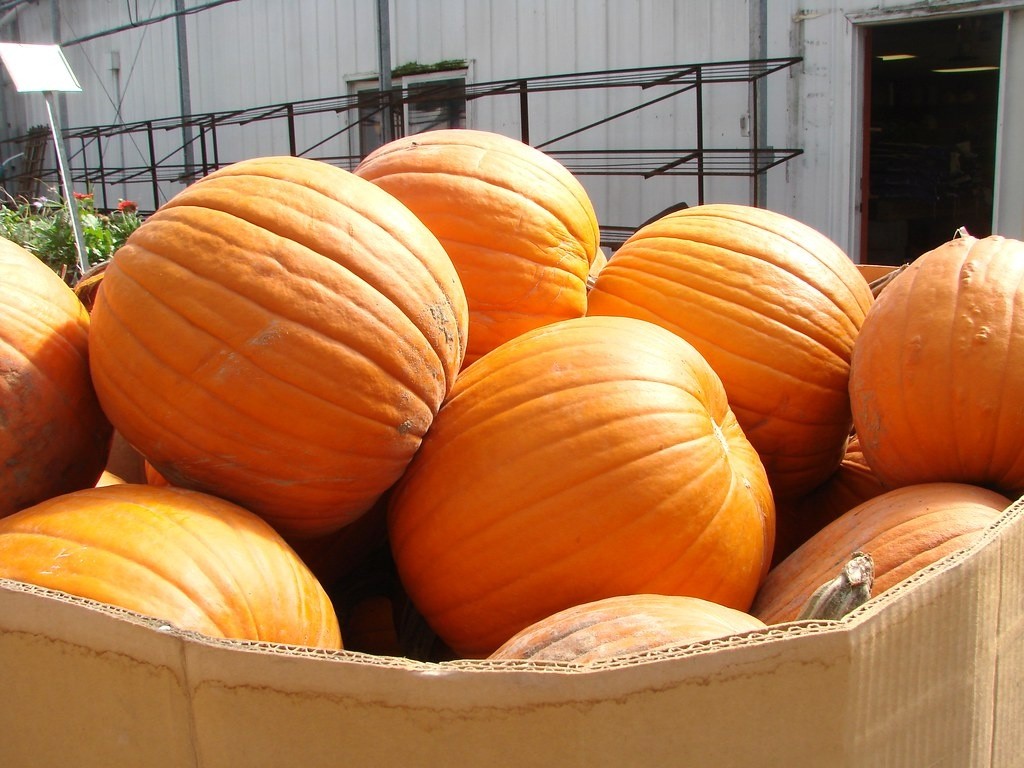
(0, 129), (1024, 663)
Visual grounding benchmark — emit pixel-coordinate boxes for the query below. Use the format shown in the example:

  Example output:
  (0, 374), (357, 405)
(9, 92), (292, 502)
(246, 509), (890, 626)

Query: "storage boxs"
(0, 263), (1024, 768)
